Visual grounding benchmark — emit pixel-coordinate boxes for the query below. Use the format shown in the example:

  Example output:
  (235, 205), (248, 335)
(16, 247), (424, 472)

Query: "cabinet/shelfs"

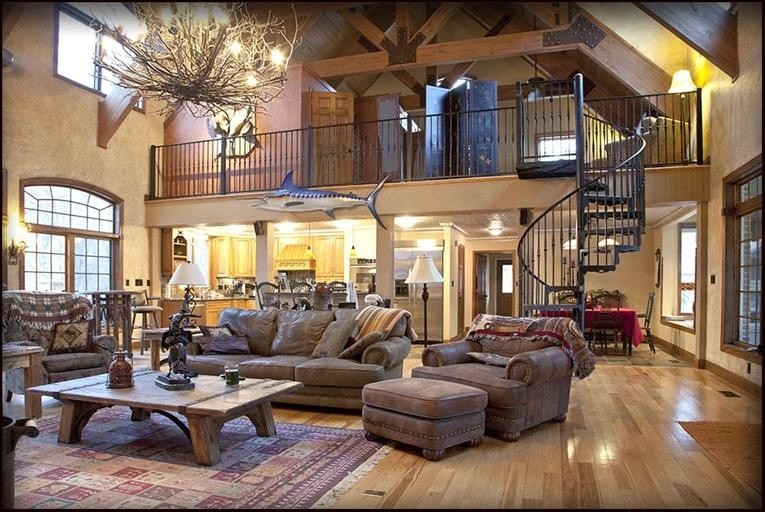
(314, 236), (344, 283)
(162, 228), (189, 276)
(208, 237), (256, 277)
(204, 299), (256, 326)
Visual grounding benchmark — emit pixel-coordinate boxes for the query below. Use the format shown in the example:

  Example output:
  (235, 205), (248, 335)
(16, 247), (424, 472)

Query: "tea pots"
(314, 281), (334, 294)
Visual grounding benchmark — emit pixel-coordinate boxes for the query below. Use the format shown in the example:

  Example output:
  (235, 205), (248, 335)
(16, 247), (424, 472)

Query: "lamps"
(668, 69), (696, 162)
(8, 220), (28, 266)
(167, 262), (209, 328)
(597, 237), (619, 247)
(306, 224), (313, 258)
(349, 224), (356, 256)
(563, 233), (577, 250)
(403, 256), (444, 349)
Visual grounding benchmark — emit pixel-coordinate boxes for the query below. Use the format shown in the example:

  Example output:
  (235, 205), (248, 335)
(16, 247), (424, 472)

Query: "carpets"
(2, 406), (394, 509)
(679, 421), (764, 496)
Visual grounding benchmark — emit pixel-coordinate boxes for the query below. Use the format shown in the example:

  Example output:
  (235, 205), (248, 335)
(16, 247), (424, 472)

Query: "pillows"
(464, 351), (508, 374)
(47, 319), (94, 353)
(199, 324), (232, 338)
(311, 320), (382, 359)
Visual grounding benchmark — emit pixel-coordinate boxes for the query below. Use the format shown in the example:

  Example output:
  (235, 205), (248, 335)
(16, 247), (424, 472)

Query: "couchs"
(182, 306), (410, 408)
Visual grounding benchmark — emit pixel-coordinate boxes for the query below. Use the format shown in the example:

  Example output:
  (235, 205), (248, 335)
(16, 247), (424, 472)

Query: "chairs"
(255, 281), (348, 310)
(129, 290), (163, 355)
(604, 117), (690, 165)
(3, 290), (117, 399)
(532, 289), (657, 359)
(411, 313), (596, 441)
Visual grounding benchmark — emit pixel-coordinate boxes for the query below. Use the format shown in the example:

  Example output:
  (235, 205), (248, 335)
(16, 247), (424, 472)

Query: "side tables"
(142, 328), (198, 369)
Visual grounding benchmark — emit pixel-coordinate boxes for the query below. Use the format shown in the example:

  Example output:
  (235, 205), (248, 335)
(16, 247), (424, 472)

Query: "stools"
(359, 375), (488, 462)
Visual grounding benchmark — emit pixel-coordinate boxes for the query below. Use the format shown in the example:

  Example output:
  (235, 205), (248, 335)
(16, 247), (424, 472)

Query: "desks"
(2, 340), (45, 420)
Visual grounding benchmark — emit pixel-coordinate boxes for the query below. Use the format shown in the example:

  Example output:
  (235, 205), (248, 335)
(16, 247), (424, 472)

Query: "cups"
(224, 364), (239, 385)
